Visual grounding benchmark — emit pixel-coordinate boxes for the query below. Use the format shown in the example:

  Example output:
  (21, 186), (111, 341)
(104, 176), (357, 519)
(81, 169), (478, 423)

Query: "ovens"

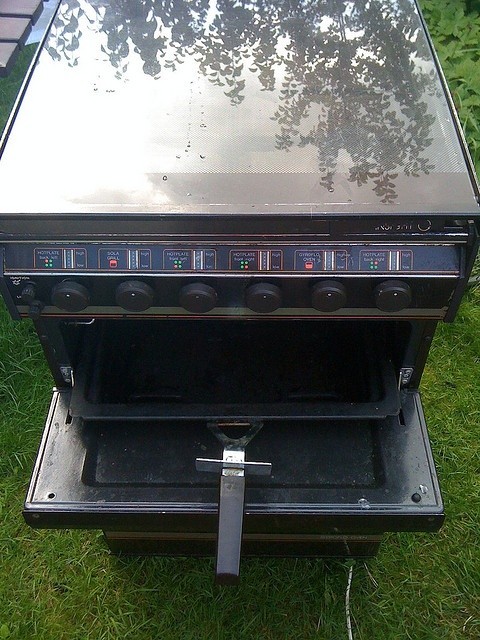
(0, 1), (478, 589)
